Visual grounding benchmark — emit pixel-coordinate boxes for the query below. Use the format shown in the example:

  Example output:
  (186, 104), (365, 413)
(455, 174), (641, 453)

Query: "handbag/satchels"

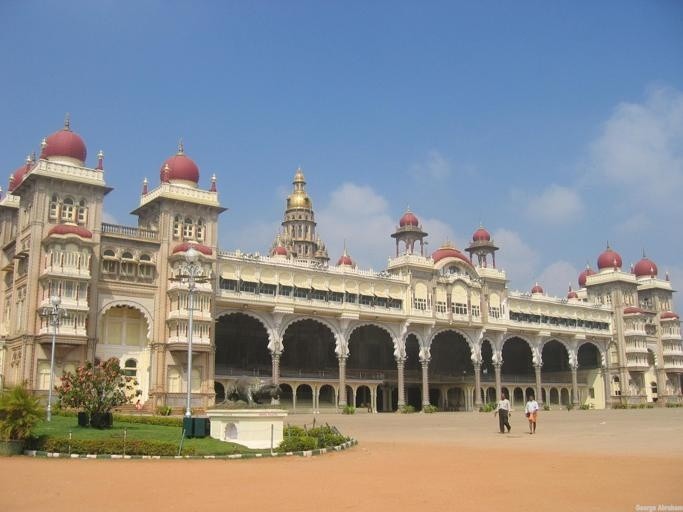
(525, 412), (530, 417)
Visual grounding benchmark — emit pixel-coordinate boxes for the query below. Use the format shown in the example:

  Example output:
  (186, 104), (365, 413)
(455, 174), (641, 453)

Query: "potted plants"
(0, 380), (47, 457)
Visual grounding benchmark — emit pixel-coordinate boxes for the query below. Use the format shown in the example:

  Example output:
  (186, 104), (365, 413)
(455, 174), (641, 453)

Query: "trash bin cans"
(183, 417), (210, 438)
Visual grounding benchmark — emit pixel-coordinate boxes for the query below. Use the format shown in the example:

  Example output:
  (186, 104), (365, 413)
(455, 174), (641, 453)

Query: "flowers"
(54, 357), (144, 413)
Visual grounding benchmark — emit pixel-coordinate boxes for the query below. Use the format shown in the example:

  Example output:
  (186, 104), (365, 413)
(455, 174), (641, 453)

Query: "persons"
(524, 395), (539, 434)
(495, 393), (512, 433)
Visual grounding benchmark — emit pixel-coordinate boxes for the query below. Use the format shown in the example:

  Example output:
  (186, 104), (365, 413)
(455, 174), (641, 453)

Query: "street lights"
(37, 295), (68, 421)
(166, 245), (216, 418)
